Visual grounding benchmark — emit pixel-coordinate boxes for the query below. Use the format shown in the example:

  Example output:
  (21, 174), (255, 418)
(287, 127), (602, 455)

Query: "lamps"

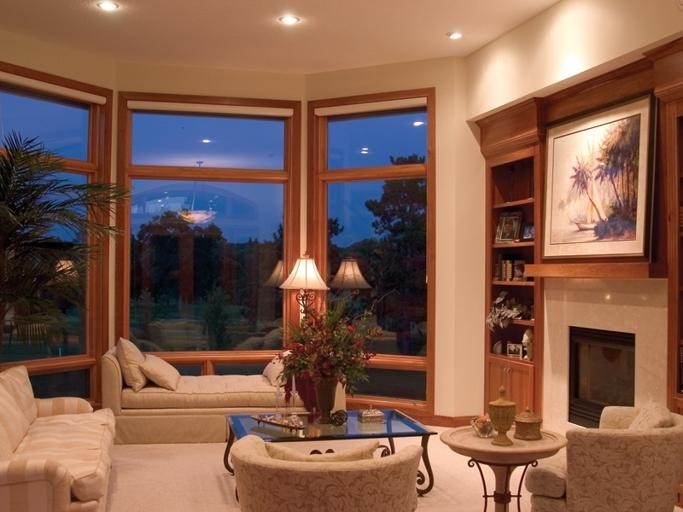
(329, 258), (370, 289)
(49, 255), (80, 285)
(178, 162), (218, 227)
(279, 256), (330, 322)
(265, 259), (283, 289)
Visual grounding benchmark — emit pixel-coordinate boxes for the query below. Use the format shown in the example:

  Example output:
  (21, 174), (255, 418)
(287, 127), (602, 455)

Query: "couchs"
(100, 345), (347, 445)
(525, 405), (683, 512)
(230, 436), (423, 512)
(0, 366), (116, 512)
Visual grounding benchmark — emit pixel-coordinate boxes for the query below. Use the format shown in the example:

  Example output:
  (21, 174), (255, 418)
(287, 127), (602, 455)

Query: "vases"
(313, 377), (339, 424)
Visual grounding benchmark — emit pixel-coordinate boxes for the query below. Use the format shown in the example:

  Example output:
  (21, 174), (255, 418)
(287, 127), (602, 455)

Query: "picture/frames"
(540, 89), (654, 260)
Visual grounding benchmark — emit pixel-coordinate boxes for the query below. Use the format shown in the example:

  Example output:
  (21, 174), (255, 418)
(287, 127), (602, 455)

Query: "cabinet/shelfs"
(483, 146), (542, 420)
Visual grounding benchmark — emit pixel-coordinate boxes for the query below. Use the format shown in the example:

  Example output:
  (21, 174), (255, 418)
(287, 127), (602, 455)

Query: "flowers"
(275, 298), (384, 386)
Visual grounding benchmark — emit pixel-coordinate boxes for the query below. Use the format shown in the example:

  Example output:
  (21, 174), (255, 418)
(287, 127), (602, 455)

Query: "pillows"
(628, 404), (672, 429)
(116, 337), (146, 393)
(138, 354), (181, 391)
(262, 349), (293, 386)
(266, 440), (378, 462)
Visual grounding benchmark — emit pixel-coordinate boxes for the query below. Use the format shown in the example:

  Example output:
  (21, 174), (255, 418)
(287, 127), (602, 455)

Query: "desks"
(441, 426), (568, 512)
